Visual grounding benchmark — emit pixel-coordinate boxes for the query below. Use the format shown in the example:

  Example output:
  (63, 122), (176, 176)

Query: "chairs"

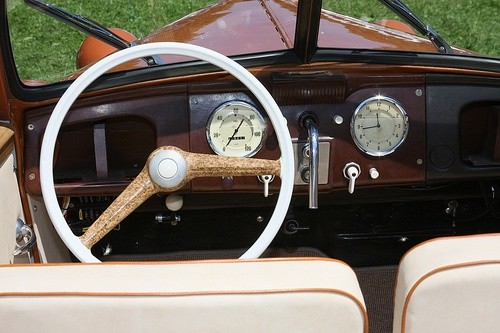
(393, 235), (500, 333)
(1, 256), (370, 333)
